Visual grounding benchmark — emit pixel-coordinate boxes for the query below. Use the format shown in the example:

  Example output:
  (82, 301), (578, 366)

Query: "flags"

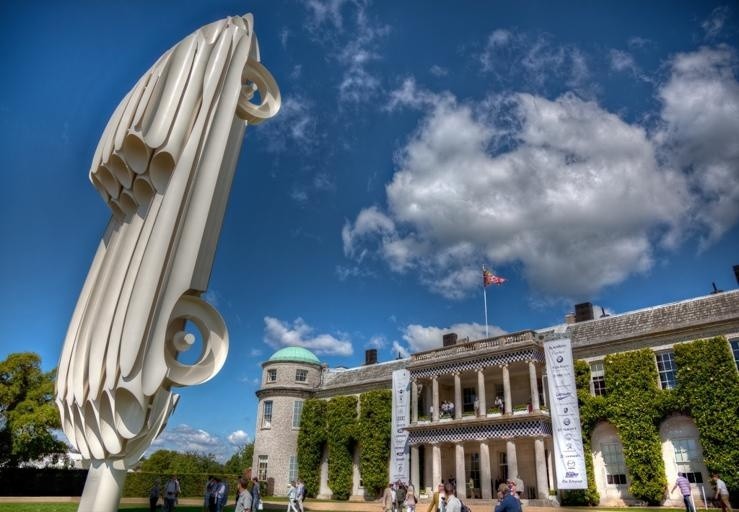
(483, 269), (511, 288)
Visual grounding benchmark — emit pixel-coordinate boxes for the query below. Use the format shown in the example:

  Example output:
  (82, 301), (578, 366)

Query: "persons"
(712, 474), (733, 512)
(494, 396), (504, 411)
(671, 472), (695, 512)
(425, 474), (474, 512)
(495, 475), (523, 512)
(203, 475), (261, 512)
(287, 480), (307, 512)
(381, 478), (417, 512)
(474, 396), (480, 418)
(149, 474), (181, 512)
(430, 399), (455, 423)
